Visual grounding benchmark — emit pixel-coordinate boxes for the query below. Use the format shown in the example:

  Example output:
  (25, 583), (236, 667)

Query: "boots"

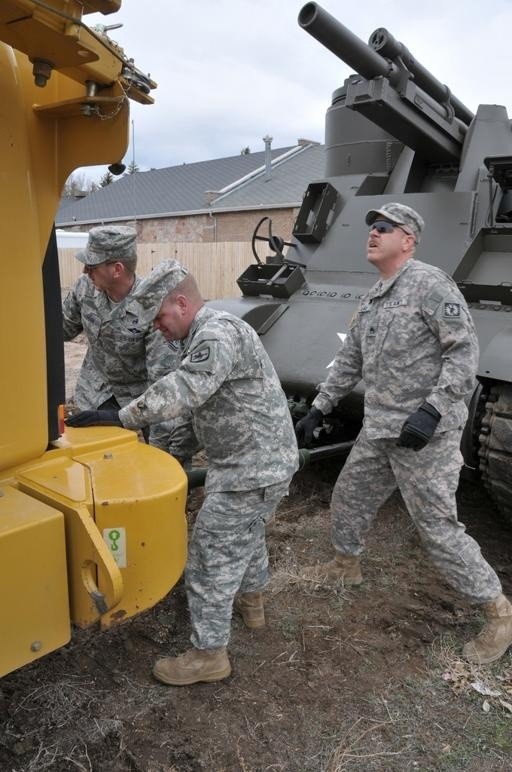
(233, 590), (266, 629)
(461, 592), (512, 665)
(296, 551), (363, 588)
(152, 644), (232, 687)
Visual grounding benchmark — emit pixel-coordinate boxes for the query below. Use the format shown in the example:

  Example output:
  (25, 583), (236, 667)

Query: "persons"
(63, 256), (302, 687)
(294, 201), (512, 665)
(63, 225), (181, 453)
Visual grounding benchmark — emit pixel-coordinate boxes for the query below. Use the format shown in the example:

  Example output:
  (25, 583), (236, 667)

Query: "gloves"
(295, 406), (324, 447)
(64, 408), (123, 428)
(396, 401), (442, 453)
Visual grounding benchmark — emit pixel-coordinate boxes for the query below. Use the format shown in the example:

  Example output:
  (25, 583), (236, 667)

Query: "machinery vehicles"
(210, 0), (512, 526)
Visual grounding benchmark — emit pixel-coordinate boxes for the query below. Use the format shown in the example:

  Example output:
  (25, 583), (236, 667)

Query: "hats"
(365, 201), (424, 245)
(130, 257), (190, 328)
(73, 225), (138, 266)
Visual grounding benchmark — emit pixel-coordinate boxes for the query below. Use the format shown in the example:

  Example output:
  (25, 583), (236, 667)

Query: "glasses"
(368, 218), (399, 235)
